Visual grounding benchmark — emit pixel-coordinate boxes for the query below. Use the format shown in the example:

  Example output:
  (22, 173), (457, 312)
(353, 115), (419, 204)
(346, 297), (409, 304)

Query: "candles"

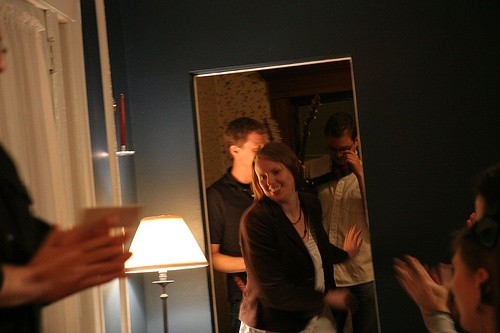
(119, 91), (127, 146)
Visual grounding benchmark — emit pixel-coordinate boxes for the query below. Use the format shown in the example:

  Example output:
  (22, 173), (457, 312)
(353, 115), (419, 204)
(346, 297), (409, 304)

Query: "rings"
(98, 274), (101, 286)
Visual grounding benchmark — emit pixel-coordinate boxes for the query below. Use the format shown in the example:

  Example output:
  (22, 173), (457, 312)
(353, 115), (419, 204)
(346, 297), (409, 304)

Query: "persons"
(393, 167), (500, 333)
(317, 112), (378, 333)
(238, 142), (363, 333)
(206, 117), (270, 333)
(0, 141), (131, 333)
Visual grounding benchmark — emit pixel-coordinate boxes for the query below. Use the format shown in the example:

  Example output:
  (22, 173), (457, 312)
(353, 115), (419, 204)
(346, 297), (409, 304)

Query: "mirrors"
(188, 52), (381, 333)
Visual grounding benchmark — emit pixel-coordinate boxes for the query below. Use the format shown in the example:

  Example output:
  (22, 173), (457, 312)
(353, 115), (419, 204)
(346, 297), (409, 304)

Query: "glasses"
(329, 137), (355, 151)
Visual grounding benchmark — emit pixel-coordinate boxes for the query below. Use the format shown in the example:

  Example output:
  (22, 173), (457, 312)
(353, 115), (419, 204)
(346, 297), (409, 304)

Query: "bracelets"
(424, 309), (454, 324)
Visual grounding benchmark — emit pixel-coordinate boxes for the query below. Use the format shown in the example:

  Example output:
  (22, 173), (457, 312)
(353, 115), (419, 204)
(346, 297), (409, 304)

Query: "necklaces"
(292, 205), (307, 239)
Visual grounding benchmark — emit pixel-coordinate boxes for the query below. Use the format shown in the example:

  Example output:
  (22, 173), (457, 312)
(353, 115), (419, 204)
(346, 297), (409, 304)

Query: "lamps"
(121, 215), (209, 333)
